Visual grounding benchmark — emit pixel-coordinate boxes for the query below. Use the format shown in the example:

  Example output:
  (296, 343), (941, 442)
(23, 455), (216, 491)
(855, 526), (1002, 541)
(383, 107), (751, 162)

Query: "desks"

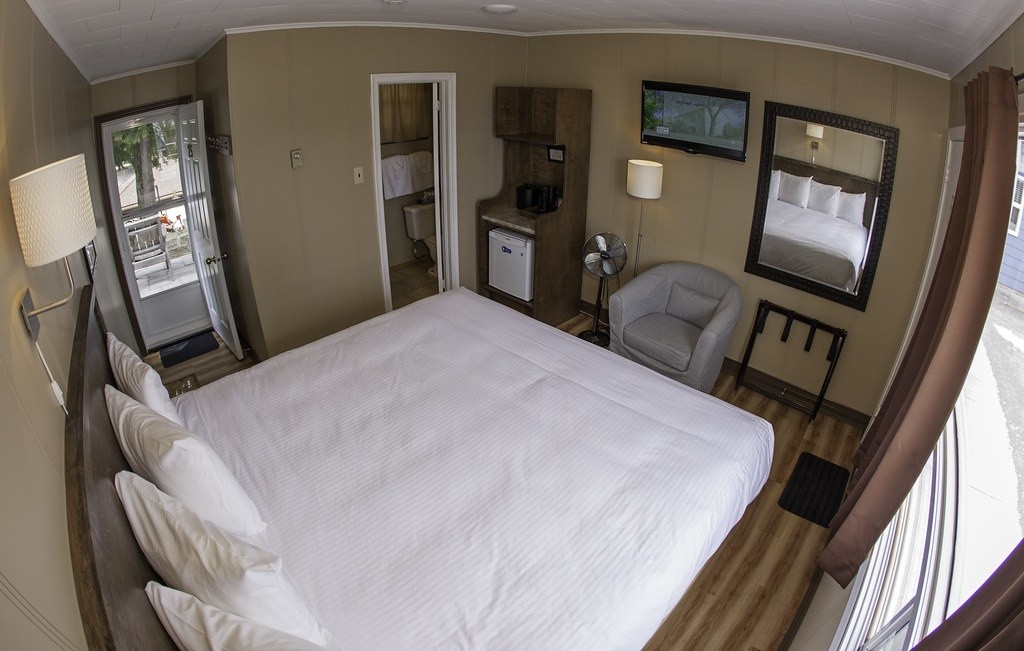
(165, 374), (200, 399)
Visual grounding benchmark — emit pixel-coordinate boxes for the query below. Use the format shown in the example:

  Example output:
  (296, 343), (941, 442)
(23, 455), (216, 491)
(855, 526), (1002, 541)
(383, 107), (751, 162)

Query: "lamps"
(8, 153), (97, 342)
(627, 159), (664, 274)
(806, 124), (824, 150)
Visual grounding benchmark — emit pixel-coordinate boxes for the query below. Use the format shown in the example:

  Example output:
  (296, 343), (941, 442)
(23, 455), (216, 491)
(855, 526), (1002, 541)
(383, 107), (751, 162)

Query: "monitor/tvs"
(640, 80), (750, 161)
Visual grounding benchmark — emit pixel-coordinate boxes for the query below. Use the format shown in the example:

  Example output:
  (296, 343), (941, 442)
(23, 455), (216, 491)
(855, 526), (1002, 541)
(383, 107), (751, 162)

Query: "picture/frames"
(83, 240), (98, 285)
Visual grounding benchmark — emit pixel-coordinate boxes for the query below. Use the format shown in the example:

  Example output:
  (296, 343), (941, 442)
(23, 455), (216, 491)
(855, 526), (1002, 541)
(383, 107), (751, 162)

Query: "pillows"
(836, 191), (867, 227)
(779, 172), (813, 210)
(106, 331), (182, 426)
(104, 384), (268, 537)
(143, 580), (323, 651)
(809, 181), (842, 217)
(769, 170), (781, 200)
(666, 282), (720, 329)
(114, 469), (325, 646)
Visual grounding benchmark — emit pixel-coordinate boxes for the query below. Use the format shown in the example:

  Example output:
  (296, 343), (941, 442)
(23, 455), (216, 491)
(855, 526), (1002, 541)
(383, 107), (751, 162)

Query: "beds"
(64, 286), (776, 651)
(759, 197), (868, 293)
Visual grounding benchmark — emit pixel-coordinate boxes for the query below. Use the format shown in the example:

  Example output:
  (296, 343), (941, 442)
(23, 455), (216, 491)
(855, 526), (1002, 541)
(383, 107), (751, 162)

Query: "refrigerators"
(488, 227), (534, 302)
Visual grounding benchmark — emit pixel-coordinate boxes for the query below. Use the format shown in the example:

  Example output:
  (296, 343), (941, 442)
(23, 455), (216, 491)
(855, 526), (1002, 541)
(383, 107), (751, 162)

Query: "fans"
(577, 231), (628, 347)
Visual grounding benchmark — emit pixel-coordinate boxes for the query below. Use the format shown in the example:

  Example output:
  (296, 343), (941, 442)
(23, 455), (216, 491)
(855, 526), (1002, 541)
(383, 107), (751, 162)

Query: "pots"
(539, 184), (562, 212)
(517, 183), (539, 209)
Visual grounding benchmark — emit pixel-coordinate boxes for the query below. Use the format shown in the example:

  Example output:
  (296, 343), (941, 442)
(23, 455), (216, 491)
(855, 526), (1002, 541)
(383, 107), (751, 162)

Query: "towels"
(381, 151), (433, 200)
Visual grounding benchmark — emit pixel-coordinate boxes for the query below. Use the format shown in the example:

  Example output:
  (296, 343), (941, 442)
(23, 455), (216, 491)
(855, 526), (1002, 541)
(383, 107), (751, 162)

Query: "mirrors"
(743, 100), (901, 313)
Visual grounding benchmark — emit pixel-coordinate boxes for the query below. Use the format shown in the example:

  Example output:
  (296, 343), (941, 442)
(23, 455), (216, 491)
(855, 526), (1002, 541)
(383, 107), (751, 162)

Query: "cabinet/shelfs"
(476, 87), (593, 328)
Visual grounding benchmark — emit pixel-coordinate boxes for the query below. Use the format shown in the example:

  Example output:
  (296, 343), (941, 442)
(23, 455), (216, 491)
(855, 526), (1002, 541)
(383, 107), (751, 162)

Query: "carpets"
(778, 452), (850, 529)
(160, 332), (220, 368)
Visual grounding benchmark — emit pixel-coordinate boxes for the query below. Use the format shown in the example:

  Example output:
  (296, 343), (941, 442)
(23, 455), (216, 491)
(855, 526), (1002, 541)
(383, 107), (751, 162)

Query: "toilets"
(403, 203), (437, 277)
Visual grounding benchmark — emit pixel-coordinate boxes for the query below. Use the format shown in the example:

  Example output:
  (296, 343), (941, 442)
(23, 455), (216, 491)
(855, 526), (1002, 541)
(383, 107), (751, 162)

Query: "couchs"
(608, 261), (743, 394)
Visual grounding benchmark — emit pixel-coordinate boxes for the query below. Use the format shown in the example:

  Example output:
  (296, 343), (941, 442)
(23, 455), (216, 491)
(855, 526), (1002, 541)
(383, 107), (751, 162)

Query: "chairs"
(125, 215), (171, 279)
